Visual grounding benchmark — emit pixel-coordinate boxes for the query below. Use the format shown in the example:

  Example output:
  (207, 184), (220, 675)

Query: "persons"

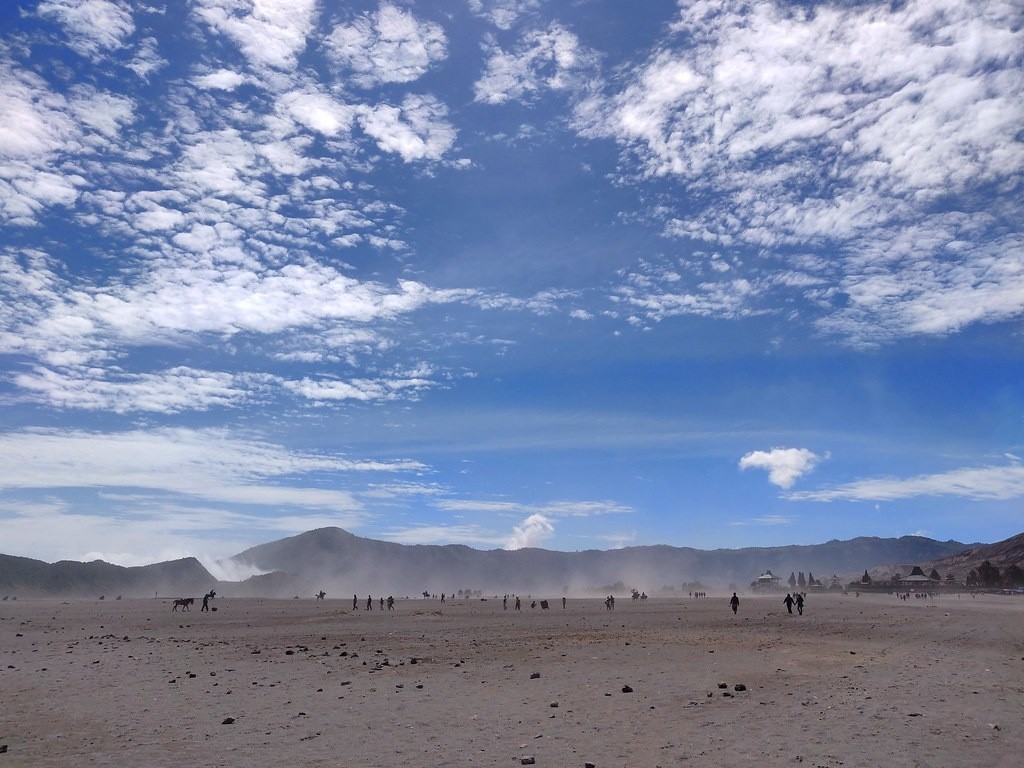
(631, 589), (646, 600)
(210, 590), (214, 598)
(730, 592), (739, 614)
(562, 597), (566, 609)
(897, 591), (927, 601)
(689, 591), (706, 600)
(503, 593), (537, 610)
(604, 595), (615, 611)
(320, 590), (323, 599)
(352, 594), (395, 610)
(425, 590), (455, 603)
(201, 594), (209, 611)
(783, 591), (806, 615)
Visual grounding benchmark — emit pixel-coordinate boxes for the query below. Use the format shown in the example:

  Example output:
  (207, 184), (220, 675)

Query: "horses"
(316, 592), (325, 600)
(172, 598), (194, 612)
